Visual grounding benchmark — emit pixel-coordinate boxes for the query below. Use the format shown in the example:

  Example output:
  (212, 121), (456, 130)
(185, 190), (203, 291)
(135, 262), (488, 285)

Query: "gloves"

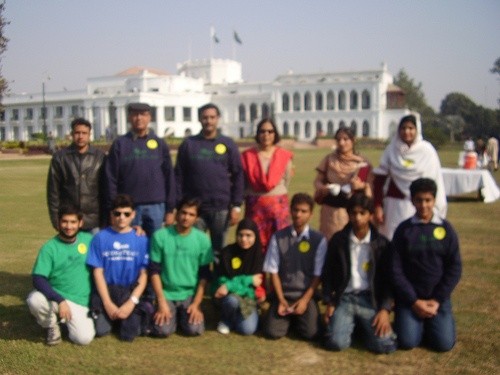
(329, 183), (340, 195)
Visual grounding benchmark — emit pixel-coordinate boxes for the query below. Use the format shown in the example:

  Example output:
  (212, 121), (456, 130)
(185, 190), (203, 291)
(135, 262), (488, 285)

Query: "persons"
(101, 102), (175, 238)
(25, 203), (145, 346)
(47, 117), (106, 236)
(85, 194), (150, 336)
(261, 192), (326, 340)
(172, 103), (244, 254)
(149, 196), (213, 337)
(315, 126), (373, 241)
(240, 118), (294, 252)
(390, 178), (462, 353)
(207, 219), (267, 336)
(457, 135), (499, 170)
(320, 193), (397, 354)
(372, 112), (448, 242)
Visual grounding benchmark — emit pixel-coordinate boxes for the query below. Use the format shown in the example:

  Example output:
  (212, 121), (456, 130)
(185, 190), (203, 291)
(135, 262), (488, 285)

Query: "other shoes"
(47, 323), (62, 344)
(217, 320), (230, 334)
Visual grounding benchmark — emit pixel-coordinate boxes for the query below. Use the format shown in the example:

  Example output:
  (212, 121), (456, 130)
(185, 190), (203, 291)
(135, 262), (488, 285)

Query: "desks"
(442, 167), (500, 203)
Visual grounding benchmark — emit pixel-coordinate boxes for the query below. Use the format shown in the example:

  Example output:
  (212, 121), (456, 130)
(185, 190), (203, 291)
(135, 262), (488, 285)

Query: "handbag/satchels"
(315, 155), (330, 205)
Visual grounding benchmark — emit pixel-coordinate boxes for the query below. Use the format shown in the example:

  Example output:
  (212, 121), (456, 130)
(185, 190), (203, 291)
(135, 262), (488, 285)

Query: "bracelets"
(232, 206), (242, 213)
(128, 295), (140, 304)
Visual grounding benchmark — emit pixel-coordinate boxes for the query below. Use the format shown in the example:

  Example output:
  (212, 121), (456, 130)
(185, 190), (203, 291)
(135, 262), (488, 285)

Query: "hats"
(128, 103), (151, 112)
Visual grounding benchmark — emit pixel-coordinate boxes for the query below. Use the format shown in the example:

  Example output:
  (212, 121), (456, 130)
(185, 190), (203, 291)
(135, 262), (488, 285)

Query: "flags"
(234, 32), (242, 44)
(213, 32), (218, 43)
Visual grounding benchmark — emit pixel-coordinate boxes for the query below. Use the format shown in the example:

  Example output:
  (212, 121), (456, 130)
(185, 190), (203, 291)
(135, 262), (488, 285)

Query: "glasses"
(259, 129), (275, 135)
(112, 211), (132, 217)
